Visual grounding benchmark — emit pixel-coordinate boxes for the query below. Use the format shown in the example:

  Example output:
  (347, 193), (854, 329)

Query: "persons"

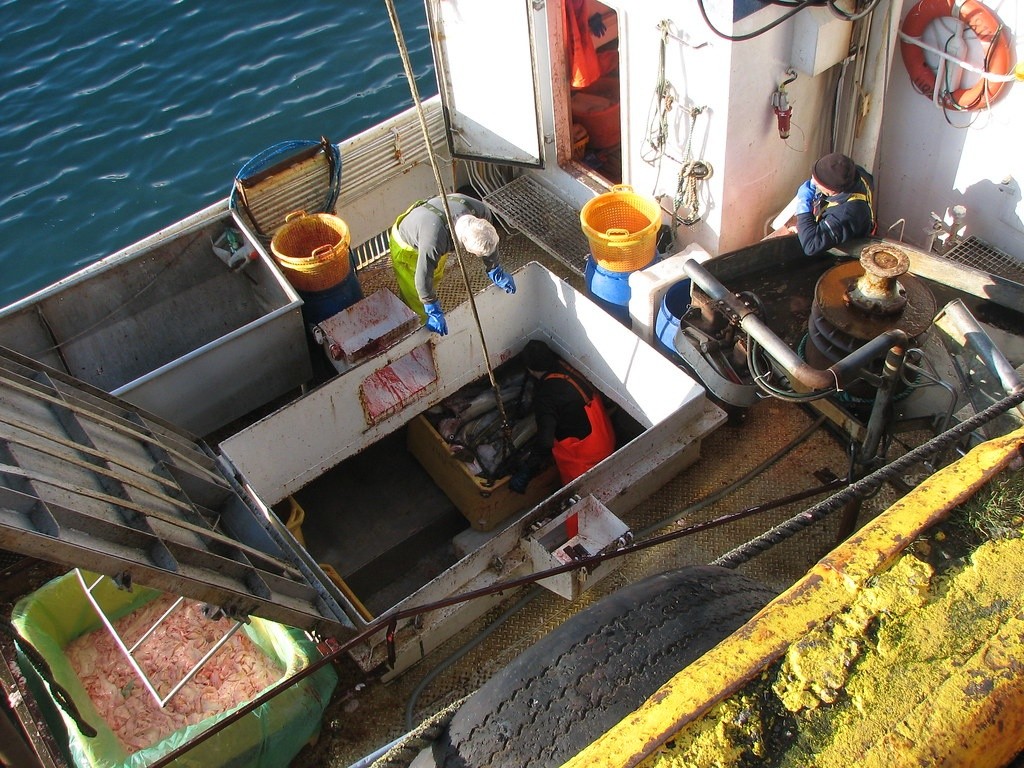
(792, 151), (874, 257)
(389, 192), (517, 337)
(507, 339), (617, 495)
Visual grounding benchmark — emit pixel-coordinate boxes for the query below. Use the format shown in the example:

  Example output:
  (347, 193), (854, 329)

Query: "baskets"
(580, 184), (662, 272)
(270, 210), (350, 292)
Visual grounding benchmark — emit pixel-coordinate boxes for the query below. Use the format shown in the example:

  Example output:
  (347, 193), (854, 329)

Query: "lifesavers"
(897, 0), (1013, 112)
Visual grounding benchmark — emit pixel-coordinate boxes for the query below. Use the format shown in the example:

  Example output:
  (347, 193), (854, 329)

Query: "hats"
(812, 153), (856, 193)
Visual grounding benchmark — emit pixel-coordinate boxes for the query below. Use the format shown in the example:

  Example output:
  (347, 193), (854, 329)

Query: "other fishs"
(439, 358), (547, 478)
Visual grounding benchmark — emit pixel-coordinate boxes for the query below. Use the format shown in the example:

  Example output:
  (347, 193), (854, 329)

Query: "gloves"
(588, 12), (607, 38)
(796, 180), (816, 215)
(424, 299), (448, 336)
(488, 265), (516, 294)
(509, 461), (535, 494)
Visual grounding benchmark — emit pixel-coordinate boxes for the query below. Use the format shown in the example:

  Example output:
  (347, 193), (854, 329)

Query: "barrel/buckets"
(655, 278), (693, 364)
(295, 256), (363, 326)
(584, 248), (661, 329)
